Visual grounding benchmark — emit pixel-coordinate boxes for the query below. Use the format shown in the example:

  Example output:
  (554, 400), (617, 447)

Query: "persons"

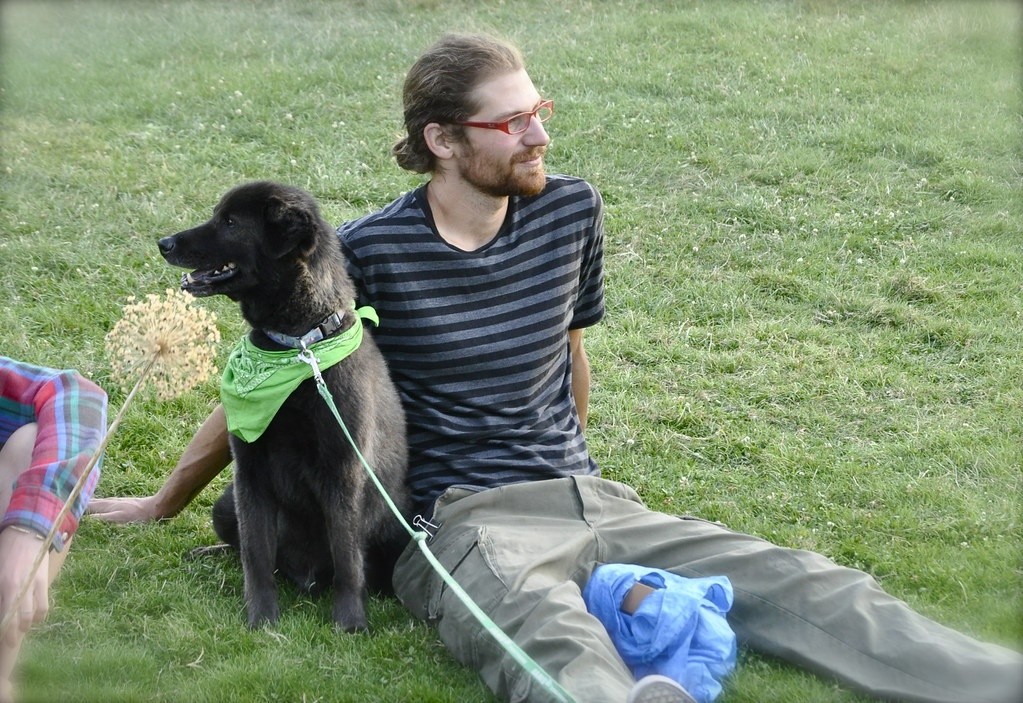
(0, 356), (107, 703)
(86, 31), (1022, 703)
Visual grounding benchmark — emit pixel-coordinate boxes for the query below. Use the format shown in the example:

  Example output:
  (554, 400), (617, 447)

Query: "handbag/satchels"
(583, 563), (736, 703)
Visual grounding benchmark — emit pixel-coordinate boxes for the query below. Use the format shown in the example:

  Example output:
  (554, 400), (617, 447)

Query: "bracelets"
(10, 525), (45, 541)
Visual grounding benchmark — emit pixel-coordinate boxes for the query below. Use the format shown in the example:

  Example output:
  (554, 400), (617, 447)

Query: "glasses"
(421, 99), (554, 135)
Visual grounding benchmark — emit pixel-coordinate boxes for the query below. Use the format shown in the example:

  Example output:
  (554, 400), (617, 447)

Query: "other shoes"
(627, 675), (697, 703)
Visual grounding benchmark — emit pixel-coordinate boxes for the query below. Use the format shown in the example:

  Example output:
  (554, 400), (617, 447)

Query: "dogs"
(156, 179), (412, 639)
